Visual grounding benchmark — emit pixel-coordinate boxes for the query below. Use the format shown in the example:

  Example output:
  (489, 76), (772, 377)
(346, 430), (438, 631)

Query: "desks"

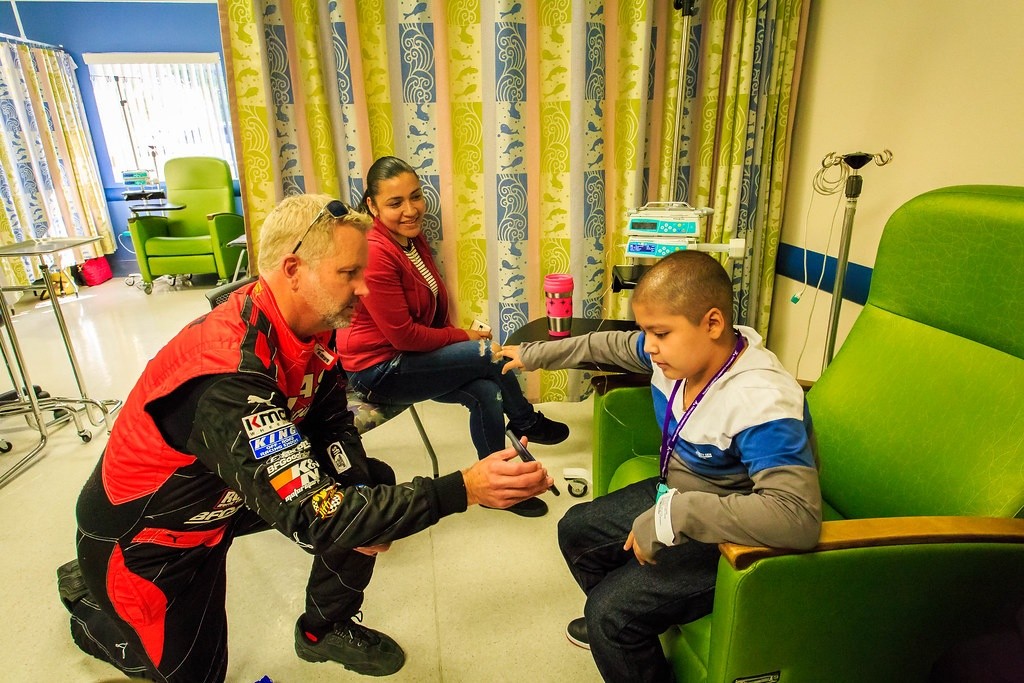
(129, 203), (187, 219)
(503, 316), (642, 499)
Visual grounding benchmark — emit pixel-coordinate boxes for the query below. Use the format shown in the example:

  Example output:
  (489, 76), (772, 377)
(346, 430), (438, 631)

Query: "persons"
(58, 193), (554, 683)
(334, 156), (570, 517)
(497, 251), (824, 683)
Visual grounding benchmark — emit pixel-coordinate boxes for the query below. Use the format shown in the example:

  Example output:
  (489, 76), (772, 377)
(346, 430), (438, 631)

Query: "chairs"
(205, 274), (443, 481)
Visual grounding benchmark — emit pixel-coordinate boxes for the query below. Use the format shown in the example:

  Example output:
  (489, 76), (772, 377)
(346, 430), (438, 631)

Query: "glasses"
(293, 200), (356, 253)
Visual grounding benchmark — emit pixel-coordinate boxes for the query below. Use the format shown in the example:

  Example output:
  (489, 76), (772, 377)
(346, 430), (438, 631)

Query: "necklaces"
(400, 237), (417, 264)
(684, 376), (689, 412)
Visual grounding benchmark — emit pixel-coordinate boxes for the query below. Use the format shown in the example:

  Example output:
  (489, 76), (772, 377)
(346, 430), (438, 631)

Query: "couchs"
(589, 184), (1024, 683)
(123, 157), (247, 294)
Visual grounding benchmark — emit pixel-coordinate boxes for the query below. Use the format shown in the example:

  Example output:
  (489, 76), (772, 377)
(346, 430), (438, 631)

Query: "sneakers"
(295, 613), (405, 677)
(56, 559), (90, 614)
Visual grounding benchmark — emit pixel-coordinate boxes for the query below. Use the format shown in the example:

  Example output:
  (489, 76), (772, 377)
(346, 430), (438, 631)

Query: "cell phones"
(469, 320), (492, 333)
(504, 430), (560, 496)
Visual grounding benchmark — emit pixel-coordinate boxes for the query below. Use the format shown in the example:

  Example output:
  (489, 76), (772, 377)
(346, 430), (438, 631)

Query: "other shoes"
(566, 616), (591, 649)
(479, 497), (549, 517)
(505, 410), (569, 445)
